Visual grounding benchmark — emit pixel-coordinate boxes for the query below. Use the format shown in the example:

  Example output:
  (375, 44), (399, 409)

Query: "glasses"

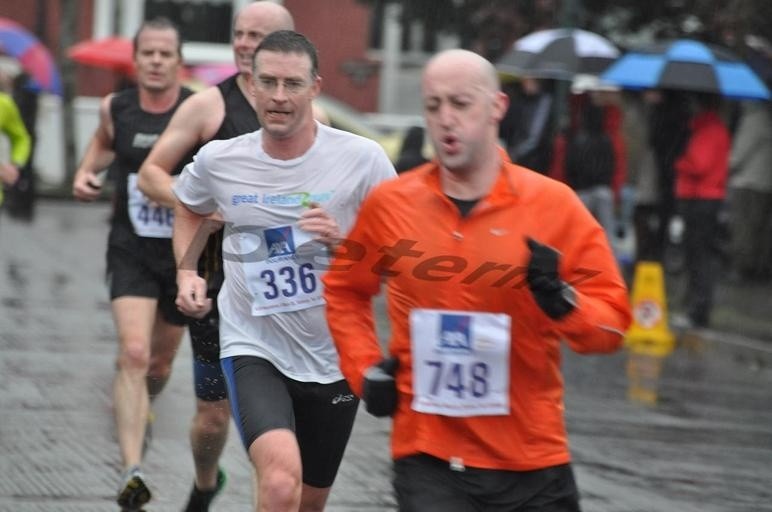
(251, 76), (315, 94)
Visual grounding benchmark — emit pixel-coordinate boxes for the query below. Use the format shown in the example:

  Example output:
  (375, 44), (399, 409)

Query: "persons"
(0, 71), (32, 208)
(138, 2), (296, 512)
(171, 29), (402, 512)
(7, 71), (38, 221)
(321, 48), (635, 512)
(71, 17), (203, 511)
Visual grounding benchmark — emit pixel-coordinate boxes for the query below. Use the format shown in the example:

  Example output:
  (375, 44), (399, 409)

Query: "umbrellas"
(500, 26), (772, 103)
(64, 37), (134, 83)
(0, 17), (67, 98)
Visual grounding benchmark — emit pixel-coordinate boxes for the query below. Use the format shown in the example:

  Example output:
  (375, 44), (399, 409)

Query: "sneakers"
(116, 462), (155, 512)
(183, 464), (228, 512)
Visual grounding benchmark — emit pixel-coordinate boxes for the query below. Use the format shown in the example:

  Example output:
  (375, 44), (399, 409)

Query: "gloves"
(516, 233), (577, 323)
(355, 354), (404, 420)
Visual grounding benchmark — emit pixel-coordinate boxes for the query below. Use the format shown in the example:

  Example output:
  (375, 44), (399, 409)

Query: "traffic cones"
(621, 259), (675, 353)
